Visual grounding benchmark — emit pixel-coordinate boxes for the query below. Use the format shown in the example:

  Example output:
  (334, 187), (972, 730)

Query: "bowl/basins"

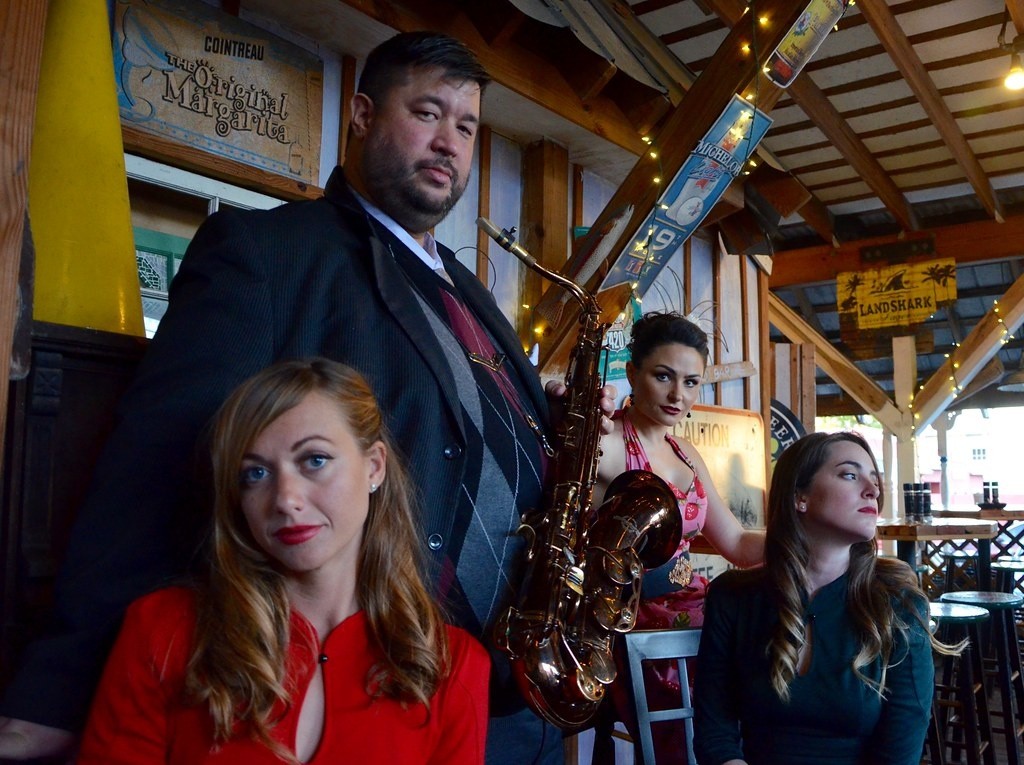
(977, 503), (1007, 510)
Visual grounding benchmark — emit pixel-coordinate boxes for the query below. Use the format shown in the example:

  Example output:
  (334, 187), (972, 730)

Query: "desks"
(876, 518), (998, 698)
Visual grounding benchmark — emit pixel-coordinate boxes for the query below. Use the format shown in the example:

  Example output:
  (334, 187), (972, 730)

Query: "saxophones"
(478, 215), (683, 733)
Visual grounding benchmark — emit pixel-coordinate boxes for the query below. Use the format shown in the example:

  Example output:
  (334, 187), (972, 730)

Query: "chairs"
(619, 629), (703, 765)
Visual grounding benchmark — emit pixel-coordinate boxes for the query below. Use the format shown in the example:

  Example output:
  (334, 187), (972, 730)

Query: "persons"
(591, 312), (770, 765)
(692, 432), (934, 765)
(0, 29), (619, 765)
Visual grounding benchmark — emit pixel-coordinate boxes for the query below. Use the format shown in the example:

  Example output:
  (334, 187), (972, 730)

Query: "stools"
(878, 551), (1024, 765)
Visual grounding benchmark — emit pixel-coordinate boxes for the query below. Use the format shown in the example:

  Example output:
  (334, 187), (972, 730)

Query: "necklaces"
(389, 243), (555, 457)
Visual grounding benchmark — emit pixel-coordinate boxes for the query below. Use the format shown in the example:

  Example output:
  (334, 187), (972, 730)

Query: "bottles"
(923, 482), (932, 525)
(983, 481), (990, 504)
(991, 481), (999, 503)
(912, 483), (923, 524)
(902, 483), (914, 525)
